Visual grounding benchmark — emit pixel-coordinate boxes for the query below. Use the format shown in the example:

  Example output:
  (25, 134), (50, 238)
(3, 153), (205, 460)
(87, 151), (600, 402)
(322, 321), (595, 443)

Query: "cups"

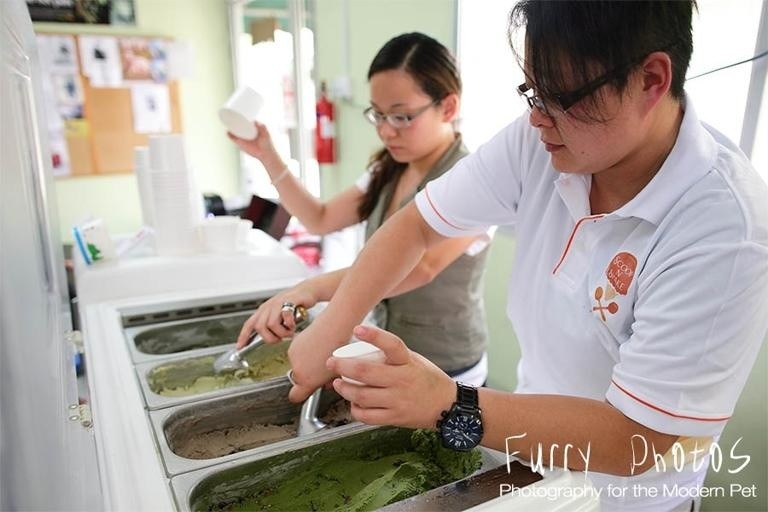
(332, 340), (387, 387)
(201, 216), (254, 260)
(133, 132), (193, 253)
(217, 86), (267, 141)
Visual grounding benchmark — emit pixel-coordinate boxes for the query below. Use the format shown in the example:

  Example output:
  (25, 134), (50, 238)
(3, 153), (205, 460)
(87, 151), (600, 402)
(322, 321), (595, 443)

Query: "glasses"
(516, 41), (677, 116)
(363, 90), (451, 130)
